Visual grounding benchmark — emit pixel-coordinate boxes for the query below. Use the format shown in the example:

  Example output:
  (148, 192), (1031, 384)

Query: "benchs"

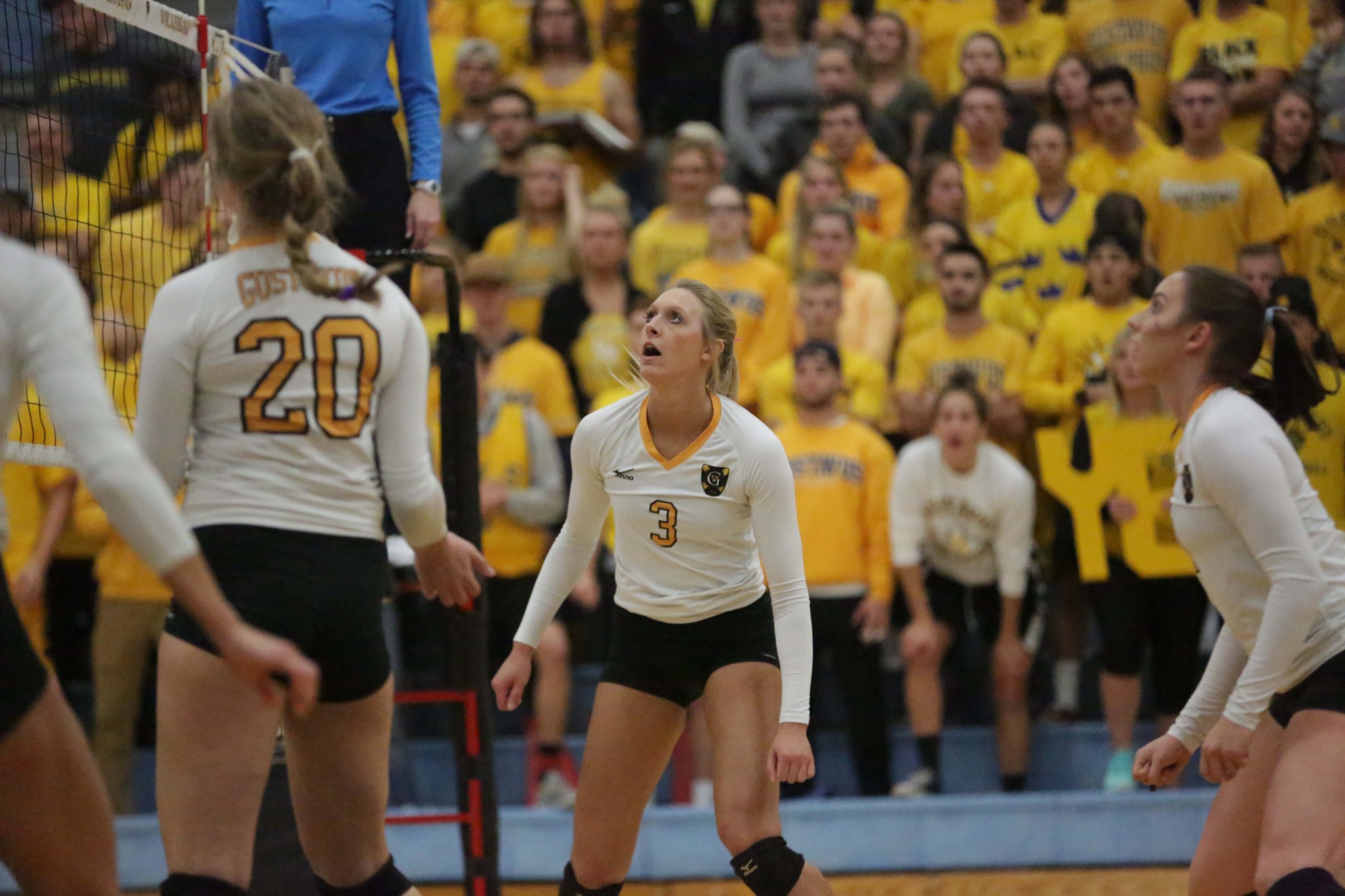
(0, 665), (1219, 896)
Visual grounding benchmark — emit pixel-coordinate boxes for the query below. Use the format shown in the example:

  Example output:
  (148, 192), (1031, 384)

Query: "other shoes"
(1101, 750), (1139, 795)
(888, 765), (944, 797)
(526, 766), (578, 812)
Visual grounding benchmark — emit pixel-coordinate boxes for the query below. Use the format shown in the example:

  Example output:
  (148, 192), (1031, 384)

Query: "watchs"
(415, 181), (441, 197)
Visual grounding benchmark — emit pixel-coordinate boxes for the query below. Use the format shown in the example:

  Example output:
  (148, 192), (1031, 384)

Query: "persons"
(0, 0), (1345, 896)
